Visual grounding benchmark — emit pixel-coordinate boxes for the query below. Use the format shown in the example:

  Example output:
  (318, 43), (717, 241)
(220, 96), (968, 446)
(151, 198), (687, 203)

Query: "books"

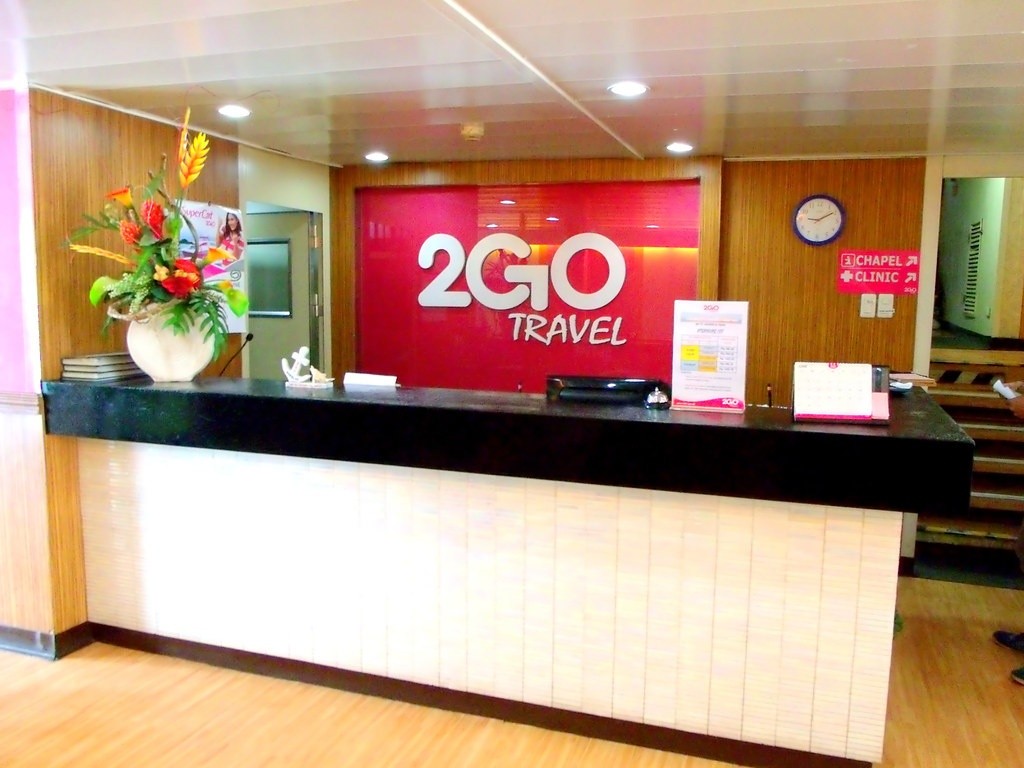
(889, 373), (936, 386)
(62, 353), (146, 382)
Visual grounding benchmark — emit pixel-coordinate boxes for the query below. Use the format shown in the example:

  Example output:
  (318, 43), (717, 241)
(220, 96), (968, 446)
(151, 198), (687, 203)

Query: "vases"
(126, 303), (215, 381)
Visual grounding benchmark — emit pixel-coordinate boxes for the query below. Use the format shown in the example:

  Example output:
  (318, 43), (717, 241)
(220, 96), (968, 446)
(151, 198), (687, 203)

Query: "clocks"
(793, 194), (846, 246)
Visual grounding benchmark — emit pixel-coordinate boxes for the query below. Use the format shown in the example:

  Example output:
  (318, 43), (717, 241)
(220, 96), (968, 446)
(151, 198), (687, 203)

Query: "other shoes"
(1011, 666), (1024, 683)
(992, 630), (1024, 653)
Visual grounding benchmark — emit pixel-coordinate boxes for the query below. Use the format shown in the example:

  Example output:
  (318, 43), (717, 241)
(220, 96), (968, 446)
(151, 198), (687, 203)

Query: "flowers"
(65, 106), (249, 360)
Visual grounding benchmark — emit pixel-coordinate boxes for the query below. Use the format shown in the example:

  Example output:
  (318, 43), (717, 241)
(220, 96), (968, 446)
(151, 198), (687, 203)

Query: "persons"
(201, 213), (245, 279)
(992, 379), (1024, 684)
